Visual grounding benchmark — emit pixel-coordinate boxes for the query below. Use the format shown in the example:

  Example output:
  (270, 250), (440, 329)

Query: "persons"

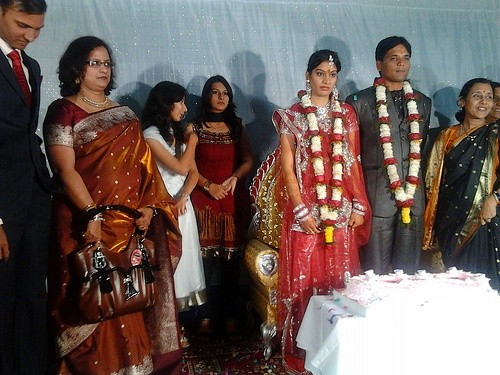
(141, 81), (208, 348)
(344, 35), (433, 275)
(484, 82), (500, 126)
(422, 78), (500, 292)
(0, 0), (61, 375)
(273, 49), (372, 375)
(186, 76), (253, 333)
(43, 36), (183, 375)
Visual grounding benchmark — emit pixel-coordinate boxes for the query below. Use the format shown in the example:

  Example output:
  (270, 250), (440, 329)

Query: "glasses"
(85, 59), (115, 69)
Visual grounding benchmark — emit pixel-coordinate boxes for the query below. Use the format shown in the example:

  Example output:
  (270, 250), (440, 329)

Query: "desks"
(296, 294), (500, 375)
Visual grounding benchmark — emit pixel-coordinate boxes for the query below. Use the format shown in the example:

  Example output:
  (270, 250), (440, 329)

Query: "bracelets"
(293, 202), (311, 222)
(204, 180), (212, 191)
(82, 203), (105, 222)
(176, 192), (189, 202)
(494, 191), (500, 204)
(353, 199), (366, 216)
(152, 208), (158, 216)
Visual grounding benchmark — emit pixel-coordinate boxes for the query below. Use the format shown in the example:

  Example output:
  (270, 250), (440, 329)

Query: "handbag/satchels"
(61, 204), (158, 323)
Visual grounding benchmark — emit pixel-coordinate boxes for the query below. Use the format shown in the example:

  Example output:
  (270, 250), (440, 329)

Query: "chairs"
(239, 147), (282, 360)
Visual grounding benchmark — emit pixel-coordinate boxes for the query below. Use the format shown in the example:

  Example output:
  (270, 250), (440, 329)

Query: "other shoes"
(224, 324), (241, 342)
(198, 323), (212, 340)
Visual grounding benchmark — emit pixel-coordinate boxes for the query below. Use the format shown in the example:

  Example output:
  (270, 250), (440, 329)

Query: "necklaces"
(373, 76), (422, 224)
(77, 93), (111, 108)
(298, 87), (343, 244)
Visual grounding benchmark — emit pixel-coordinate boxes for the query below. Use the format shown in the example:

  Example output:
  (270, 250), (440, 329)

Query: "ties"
(7, 50), (32, 110)
